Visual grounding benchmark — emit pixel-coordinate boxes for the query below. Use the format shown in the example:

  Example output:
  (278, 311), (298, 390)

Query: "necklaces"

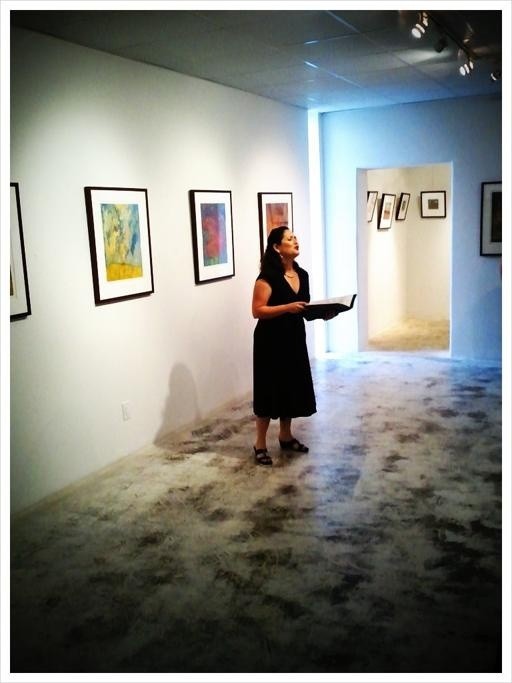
(281, 268), (302, 280)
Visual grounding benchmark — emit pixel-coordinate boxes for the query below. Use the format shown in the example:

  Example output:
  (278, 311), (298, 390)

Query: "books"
(300, 292), (357, 319)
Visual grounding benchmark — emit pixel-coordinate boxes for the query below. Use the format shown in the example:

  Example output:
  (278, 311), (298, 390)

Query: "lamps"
(411, 10), (501, 83)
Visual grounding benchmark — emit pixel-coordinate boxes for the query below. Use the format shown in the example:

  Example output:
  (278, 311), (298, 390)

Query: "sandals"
(253, 446), (272, 464)
(279, 438), (309, 452)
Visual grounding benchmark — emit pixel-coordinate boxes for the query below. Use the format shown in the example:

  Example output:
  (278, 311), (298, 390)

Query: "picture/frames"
(187, 188), (236, 285)
(366, 189), (447, 230)
(257, 191), (294, 260)
(84, 185), (156, 306)
(10, 181), (32, 321)
(479, 180), (502, 257)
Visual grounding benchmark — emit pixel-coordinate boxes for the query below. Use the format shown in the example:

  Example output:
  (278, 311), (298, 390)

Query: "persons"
(252, 226), (340, 463)
